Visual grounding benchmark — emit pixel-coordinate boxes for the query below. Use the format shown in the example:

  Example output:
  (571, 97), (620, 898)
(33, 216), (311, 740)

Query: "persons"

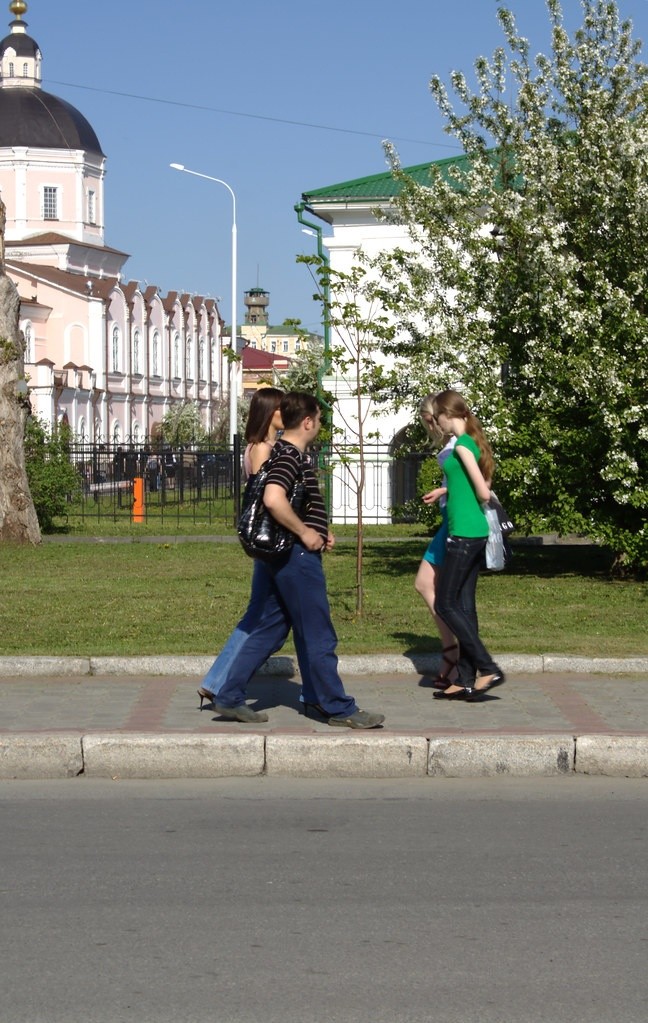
(197, 388), (386, 730)
(91, 439), (198, 493)
(414, 389), (507, 703)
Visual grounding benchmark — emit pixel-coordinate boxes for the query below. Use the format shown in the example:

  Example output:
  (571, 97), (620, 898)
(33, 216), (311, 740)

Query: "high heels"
(431, 645), (458, 682)
(197, 690), (216, 710)
(299, 692), (331, 718)
(434, 665), (465, 688)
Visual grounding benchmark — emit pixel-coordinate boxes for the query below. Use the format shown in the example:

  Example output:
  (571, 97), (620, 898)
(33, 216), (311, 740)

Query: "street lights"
(169, 162), (238, 451)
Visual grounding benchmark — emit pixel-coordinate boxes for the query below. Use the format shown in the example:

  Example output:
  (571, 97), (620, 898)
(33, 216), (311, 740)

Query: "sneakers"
(328, 708), (385, 729)
(215, 703), (268, 723)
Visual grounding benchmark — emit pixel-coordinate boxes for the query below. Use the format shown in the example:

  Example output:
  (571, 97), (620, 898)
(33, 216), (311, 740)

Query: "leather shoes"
(433, 689), (471, 699)
(464, 671), (504, 700)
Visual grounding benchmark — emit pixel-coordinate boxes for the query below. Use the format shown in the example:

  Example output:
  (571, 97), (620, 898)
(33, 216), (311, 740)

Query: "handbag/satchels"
(237, 446), (306, 562)
(482, 500), (506, 572)
(489, 498), (515, 538)
